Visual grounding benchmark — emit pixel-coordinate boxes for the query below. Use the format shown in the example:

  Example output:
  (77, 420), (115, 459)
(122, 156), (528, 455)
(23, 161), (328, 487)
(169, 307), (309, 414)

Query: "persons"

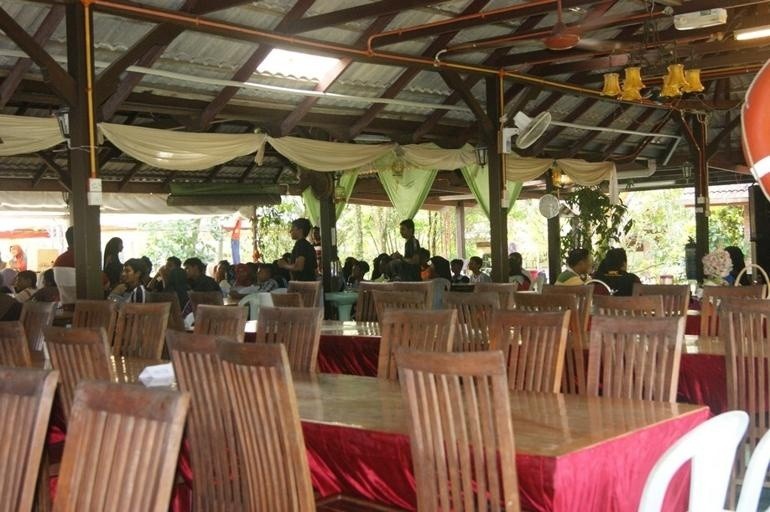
(554, 248), (591, 285)
(103, 216), (318, 312)
(590, 248), (642, 296)
(0, 226), (76, 323)
(337, 218), (534, 291)
(723, 247), (749, 286)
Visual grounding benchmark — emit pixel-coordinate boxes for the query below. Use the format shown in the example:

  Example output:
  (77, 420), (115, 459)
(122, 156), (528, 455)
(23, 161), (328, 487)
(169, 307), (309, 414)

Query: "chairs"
(2, 318), (65, 510)
(3, 282), (766, 378)
(212, 336), (403, 510)
(583, 314), (688, 401)
(716, 294), (769, 511)
(1, 366), (58, 509)
(51, 378), (193, 510)
(38, 323), (118, 425)
(391, 342), (522, 511)
(163, 326), (239, 500)
(487, 307), (572, 393)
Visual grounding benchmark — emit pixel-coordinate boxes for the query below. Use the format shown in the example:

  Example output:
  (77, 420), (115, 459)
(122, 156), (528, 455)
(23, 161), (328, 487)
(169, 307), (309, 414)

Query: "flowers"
(702, 249), (733, 279)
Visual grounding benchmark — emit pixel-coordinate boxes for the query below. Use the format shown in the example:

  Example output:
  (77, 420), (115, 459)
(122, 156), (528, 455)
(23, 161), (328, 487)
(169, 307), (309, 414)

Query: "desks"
(168, 320), (768, 427)
(62, 354), (711, 509)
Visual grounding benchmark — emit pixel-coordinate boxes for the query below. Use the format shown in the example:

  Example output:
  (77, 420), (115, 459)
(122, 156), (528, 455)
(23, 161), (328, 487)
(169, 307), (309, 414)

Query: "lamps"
(54, 106), (71, 151)
(674, 8), (727, 31)
(601, 0), (705, 102)
(734, 25), (770, 41)
(682, 157), (693, 180)
(61, 190), (71, 209)
(474, 142), (488, 169)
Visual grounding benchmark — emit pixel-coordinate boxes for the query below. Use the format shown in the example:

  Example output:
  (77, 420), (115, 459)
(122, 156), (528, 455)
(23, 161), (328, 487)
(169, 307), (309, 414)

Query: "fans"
(502, 111), (552, 153)
(538, 194), (561, 219)
(476, 0), (625, 53)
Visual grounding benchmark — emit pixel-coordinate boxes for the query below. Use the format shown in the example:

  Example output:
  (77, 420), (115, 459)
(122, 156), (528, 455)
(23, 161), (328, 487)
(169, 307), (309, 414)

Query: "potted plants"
(685, 235), (696, 252)
